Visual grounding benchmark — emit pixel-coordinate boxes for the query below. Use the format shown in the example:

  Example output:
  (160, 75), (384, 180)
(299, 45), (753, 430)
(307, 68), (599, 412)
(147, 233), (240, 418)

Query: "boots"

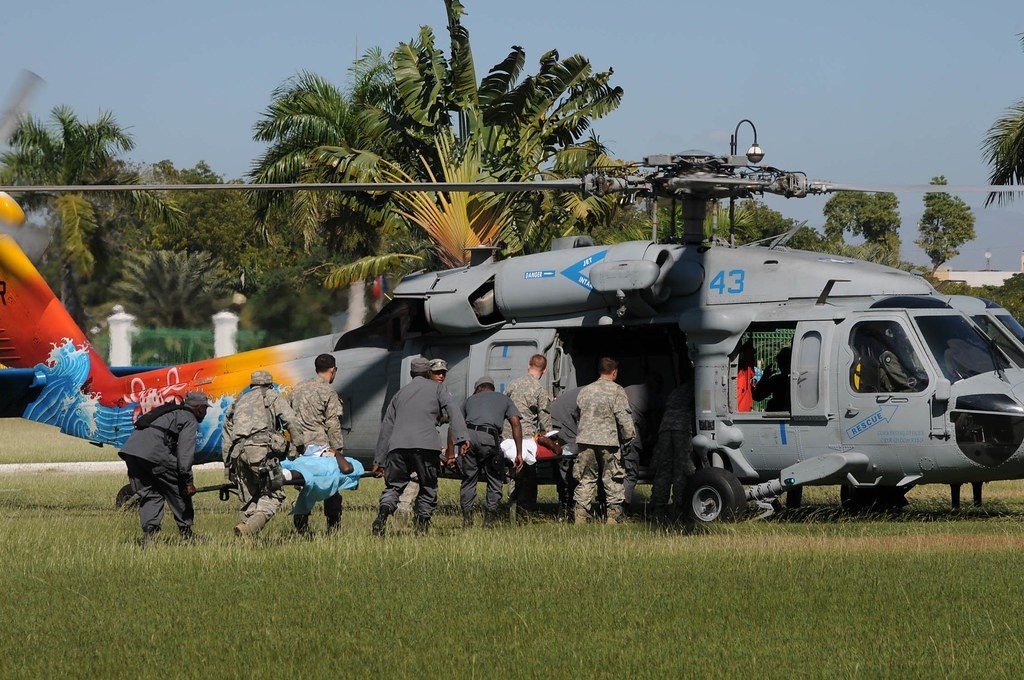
(482, 512), (495, 529)
(294, 515), (313, 537)
(372, 506), (390, 536)
(413, 523), (427, 535)
(326, 515), (341, 534)
(234, 512), (266, 541)
(465, 511), (472, 529)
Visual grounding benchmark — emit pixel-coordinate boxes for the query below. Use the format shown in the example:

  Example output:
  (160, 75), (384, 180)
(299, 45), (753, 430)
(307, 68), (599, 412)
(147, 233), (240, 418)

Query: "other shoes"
(143, 533), (168, 544)
(178, 527), (206, 544)
(605, 518), (618, 524)
(574, 517), (587, 525)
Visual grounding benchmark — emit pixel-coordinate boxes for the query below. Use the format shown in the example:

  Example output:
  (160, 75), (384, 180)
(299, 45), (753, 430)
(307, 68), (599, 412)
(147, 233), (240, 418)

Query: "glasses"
(333, 366), (337, 371)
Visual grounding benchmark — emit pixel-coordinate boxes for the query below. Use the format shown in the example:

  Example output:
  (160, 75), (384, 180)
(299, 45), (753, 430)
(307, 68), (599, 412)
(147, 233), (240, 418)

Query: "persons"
(447, 377), (523, 526)
(290, 447), (353, 479)
(752, 347), (791, 411)
(503, 354), (690, 524)
(877, 322), (928, 392)
(222, 371), (306, 541)
(287, 354), (344, 533)
(372, 358), (471, 535)
(116, 391), (212, 542)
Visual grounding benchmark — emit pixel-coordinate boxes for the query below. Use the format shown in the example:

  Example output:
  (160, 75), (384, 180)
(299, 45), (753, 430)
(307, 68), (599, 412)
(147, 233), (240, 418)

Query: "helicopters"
(0, 148), (1024, 531)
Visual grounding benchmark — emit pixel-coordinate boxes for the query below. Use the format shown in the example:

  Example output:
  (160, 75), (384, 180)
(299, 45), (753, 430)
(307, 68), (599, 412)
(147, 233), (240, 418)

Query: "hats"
(410, 357), (431, 373)
(474, 376), (495, 392)
(250, 370), (273, 389)
(429, 359), (448, 371)
(184, 392), (211, 407)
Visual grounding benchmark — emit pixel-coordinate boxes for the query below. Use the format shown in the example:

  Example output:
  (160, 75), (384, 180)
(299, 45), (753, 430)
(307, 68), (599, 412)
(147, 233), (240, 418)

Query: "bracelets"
(448, 457), (455, 459)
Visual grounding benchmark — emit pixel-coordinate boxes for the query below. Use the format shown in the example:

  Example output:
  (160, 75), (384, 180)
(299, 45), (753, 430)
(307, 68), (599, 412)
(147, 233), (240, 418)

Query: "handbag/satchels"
(268, 430), (286, 456)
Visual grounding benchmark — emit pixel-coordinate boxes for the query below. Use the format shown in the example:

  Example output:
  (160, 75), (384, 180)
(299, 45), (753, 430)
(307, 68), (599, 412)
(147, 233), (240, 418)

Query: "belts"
(467, 423), (496, 437)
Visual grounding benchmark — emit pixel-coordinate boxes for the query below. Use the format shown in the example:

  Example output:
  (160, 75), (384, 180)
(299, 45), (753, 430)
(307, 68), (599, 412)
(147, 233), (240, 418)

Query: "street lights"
(726, 118), (771, 242)
